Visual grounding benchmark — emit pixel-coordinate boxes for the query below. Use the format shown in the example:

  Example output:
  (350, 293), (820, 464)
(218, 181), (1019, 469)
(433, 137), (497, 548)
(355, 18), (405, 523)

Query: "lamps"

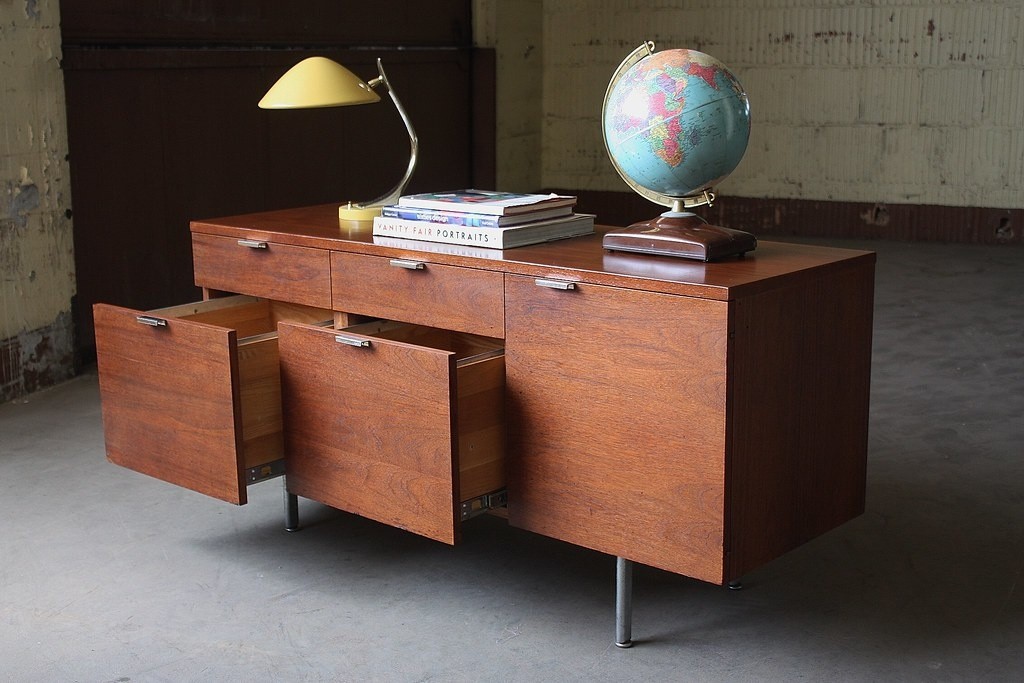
(258, 57), (419, 219)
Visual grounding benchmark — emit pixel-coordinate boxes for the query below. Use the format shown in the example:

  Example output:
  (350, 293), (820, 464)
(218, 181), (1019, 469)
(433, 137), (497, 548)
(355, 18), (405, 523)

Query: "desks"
(94, 199), (876, 648)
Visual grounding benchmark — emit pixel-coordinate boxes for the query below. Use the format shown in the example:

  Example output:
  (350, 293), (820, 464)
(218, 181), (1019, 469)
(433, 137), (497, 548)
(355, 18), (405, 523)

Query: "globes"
(599, 41), (759, 262)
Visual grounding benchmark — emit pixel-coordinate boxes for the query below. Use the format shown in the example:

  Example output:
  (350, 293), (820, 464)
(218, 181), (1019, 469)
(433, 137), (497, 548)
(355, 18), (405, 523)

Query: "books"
(373, 216), (597, 250)
(381, 188), (577, 227)
